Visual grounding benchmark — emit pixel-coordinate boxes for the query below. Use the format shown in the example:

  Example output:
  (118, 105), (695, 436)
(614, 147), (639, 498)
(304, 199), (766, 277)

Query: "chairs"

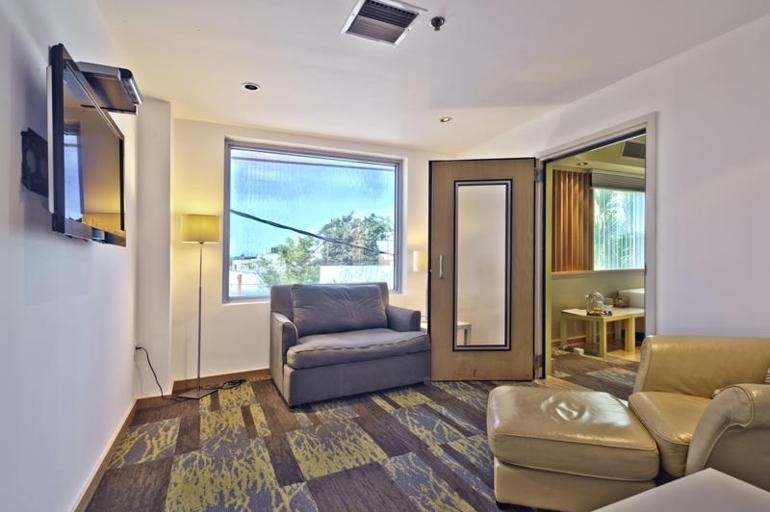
(630, 337), (770, 488)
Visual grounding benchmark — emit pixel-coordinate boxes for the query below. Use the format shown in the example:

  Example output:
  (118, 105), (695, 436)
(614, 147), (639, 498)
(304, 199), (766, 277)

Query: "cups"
(603, 297), (612, 316)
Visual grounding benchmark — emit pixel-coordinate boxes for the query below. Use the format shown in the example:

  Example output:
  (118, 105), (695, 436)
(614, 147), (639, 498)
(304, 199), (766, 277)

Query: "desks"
(588, 465), (770, 510)
(420, 319), (472, 348)
(561, 304), (645, 355)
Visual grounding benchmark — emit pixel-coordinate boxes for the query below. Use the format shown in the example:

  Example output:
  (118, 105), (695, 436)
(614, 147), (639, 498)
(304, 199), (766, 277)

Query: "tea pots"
(585, 290), (602, 316)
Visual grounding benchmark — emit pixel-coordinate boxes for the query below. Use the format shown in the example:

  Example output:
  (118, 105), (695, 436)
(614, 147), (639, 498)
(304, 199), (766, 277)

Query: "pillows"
(290, 284), (388, 337)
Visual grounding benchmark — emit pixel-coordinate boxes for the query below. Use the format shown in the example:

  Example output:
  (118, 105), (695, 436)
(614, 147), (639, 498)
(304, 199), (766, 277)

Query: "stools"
(486, 385), (660, 509)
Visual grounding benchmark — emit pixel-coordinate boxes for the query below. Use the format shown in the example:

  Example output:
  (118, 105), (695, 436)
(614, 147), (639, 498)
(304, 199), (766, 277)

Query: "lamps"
(176, 213), (221, 401)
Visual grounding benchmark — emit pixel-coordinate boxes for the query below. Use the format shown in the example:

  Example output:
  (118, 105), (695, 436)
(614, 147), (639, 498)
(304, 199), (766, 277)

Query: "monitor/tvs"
(46, 42), (127, 248)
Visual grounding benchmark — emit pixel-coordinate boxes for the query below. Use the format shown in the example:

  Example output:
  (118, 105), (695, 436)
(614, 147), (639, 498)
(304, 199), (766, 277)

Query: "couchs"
(269, 282), (432, 408)
